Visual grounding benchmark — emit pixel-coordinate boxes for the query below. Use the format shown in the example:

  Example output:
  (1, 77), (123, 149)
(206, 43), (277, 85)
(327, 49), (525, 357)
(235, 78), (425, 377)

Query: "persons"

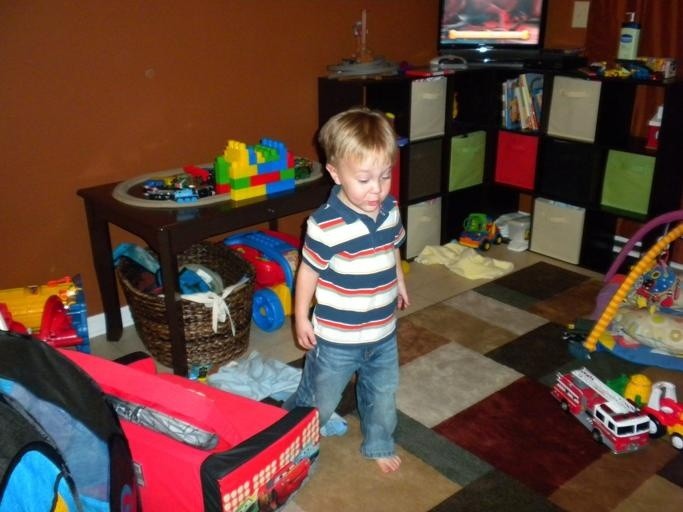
(280, 109), (409, 474)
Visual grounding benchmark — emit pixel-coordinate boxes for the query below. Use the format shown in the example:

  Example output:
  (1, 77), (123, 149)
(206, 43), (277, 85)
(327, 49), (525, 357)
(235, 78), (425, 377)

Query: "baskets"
(115, 241), (253, 370)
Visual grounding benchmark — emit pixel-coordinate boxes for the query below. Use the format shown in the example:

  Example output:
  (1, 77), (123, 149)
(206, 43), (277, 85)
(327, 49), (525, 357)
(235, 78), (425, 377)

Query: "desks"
(77, 160), (335, 377)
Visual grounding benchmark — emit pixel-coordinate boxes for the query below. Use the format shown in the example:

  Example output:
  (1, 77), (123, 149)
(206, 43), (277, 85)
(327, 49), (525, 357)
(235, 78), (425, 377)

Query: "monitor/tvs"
(435, 0), (548, 70)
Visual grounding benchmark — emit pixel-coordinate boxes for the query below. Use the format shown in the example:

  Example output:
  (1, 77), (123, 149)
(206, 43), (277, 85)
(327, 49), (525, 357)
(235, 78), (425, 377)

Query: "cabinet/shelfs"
(317, 56), (683, 280)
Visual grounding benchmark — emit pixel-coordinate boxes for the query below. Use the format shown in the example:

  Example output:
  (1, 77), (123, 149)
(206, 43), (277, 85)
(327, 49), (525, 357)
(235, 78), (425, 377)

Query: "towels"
(206, 351), (348, 437)
(415, 243), (515, 281)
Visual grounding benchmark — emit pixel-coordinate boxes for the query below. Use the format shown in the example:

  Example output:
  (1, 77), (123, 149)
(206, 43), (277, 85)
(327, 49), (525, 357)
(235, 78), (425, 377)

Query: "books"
(502, 74), (543, 131)
